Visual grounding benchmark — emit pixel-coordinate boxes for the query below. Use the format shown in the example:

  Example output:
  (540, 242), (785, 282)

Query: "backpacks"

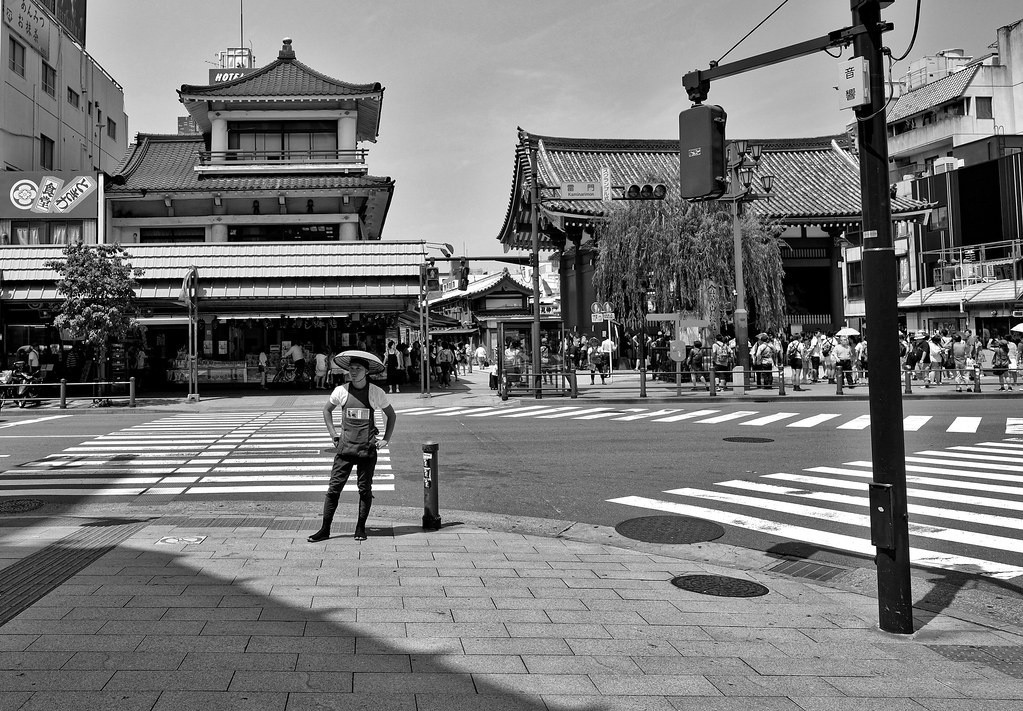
(693, 353), (701, 365)
(788, 348), (798, 358)
(719, 349), (727, 363)
(995, 352), (1009, 367)
(822, 345), (832, 356)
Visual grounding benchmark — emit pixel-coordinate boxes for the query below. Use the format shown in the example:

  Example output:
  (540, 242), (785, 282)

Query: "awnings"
(897, 278), (1023, 313)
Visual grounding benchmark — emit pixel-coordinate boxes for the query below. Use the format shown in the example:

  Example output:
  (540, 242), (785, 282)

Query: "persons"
(26, 341), (41, 380)
(257, 346), (269, 390)
(131, 344), (147, 389)
(307, 350), (397, 542)
(66, 344), (87, 392)
(281, 322), (1023, 393)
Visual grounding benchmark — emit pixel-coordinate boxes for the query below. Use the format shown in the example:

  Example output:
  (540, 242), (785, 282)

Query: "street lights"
(710, 137), (777, 390)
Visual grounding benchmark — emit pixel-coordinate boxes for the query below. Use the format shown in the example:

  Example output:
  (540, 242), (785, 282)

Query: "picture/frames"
(893, 236), (909, 256)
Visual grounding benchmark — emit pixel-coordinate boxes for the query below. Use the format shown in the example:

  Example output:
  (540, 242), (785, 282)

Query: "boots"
(794, 385), (796, 391)
(796, 386), (805, 391)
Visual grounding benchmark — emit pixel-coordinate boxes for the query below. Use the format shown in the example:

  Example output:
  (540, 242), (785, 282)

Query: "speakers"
(678, 104), (727, 202)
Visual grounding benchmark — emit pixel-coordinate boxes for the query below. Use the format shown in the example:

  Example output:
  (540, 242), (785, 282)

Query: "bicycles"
(272, 357), (311, 387)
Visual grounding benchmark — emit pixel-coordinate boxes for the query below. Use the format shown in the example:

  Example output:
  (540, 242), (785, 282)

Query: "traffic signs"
(601, 168), (612, 203)
(561, 181), (602, 199)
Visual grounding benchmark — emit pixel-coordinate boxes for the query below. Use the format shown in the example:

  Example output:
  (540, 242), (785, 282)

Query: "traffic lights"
(624, 183), (667, 199)
(426, 267), (440, 292)
(457, 260), (470, 291)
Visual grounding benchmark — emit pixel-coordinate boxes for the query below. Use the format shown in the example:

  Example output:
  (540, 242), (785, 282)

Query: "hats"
(914, 334), (925, 340)
(334, 351), (385, 375)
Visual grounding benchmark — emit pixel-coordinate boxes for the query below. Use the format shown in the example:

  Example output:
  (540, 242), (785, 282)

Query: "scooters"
(0, 360), (44, 408)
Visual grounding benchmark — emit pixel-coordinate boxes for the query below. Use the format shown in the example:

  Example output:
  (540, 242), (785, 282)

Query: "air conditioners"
(955, 263), (982, 281)
(226, 148), (245, 162)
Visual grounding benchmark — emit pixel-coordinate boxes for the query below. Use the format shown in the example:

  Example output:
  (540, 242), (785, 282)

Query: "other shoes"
(956, 387), (962, 392)
(706, 386), (709, 391)
(1008, 386), (1013, 391)
(690, 387), (699, 392)
(967, 387), (972, 392)
(999, 387), (1006, 392)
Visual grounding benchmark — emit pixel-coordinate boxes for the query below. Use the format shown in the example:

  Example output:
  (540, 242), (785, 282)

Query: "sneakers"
(308, 525), (333, 543)
(354, 523), (367, 541)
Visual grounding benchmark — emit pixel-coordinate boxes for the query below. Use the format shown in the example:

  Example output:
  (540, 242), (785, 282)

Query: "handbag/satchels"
(944, 355), (956, 369)
